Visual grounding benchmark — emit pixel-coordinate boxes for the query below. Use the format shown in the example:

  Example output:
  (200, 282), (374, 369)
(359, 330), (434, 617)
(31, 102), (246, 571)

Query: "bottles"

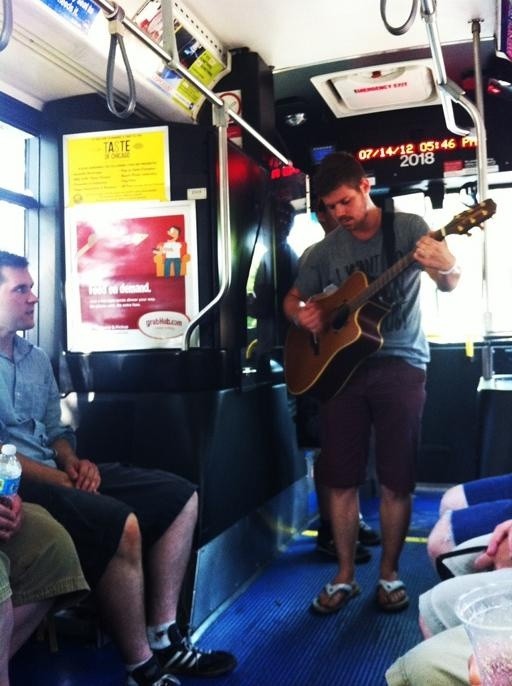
(1, 443), (23, 509)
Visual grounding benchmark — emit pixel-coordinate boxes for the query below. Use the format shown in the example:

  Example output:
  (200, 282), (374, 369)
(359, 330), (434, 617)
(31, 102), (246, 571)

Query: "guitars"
(284, 199), (496, 406)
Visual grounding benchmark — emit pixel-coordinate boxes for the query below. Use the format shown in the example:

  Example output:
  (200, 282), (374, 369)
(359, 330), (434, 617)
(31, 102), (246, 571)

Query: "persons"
(0, 493), (90, 686)
(295, 196), (382, 564)
(418, 519), (512, 639)
(245, 197), (299, 368)
(0, 252), (237, 686)
(385, 611), (512, 686)
(427, 469), (512, 574)
(283, 151), (459, 611)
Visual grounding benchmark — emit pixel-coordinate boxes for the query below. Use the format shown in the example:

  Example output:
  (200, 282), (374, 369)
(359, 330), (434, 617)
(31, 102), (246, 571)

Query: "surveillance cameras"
(285, 113), (306, 127)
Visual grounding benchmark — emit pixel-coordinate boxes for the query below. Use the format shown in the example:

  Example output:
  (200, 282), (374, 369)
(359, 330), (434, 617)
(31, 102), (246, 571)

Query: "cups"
(455, 583), (511, 686)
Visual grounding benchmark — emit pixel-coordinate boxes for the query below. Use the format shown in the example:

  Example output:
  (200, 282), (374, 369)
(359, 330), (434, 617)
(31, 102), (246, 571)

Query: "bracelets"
(438, 255), (459, 275)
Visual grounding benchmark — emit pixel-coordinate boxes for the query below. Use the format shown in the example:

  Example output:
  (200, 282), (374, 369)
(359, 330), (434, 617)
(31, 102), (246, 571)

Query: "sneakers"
(151, 621), (237, 678)
(315, 528), (372, 563)
(357, 520), (381, 546)
(124, 653), (183, 686)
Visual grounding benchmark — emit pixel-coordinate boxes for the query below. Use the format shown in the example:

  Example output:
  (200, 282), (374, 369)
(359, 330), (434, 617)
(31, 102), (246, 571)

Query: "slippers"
(311, 582), (362, 616)
(378, 579), (410, 612)
